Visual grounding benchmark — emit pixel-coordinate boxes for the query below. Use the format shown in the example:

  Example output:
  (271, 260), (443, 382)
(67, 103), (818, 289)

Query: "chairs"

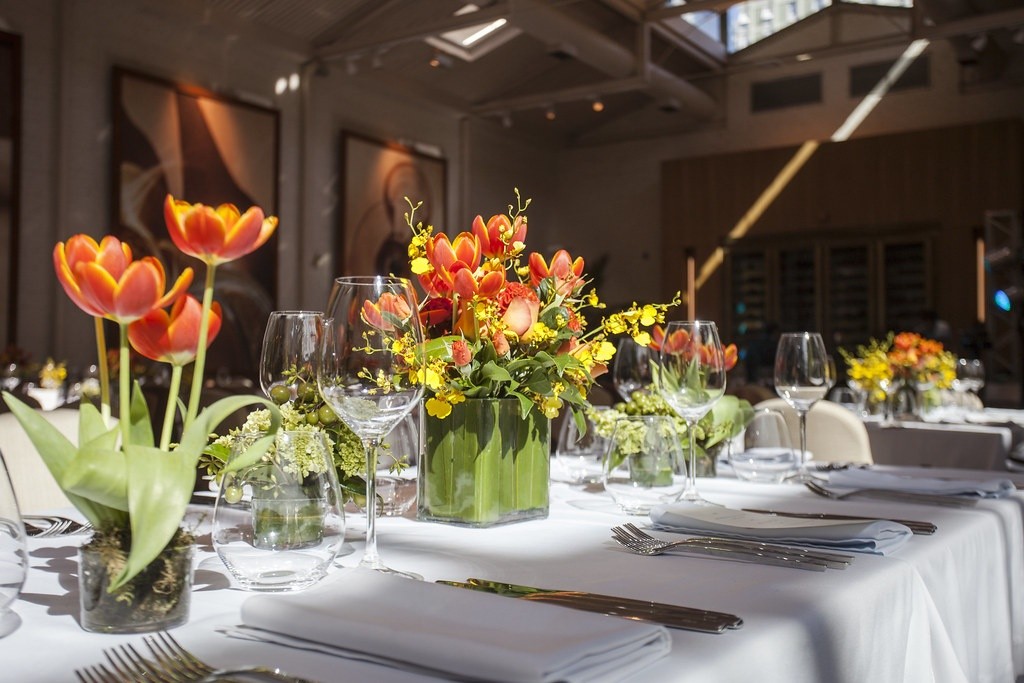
(745, 397), (872, 467)
(0, 408), (122, 525)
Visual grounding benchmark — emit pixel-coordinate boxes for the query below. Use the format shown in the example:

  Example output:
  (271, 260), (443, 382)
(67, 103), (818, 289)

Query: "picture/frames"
(107, 66), (282, 413)
(335, 129), (449, 282)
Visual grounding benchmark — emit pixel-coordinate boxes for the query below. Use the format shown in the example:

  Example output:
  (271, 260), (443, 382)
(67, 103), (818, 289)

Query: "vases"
(79, 543), (193, 635)
(888, 388), (919, 421)
(688, 438), (724, 477)
(418, 396), (551, 530)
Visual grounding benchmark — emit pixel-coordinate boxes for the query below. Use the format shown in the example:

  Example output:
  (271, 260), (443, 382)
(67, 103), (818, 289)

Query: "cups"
(340, 397), (419, 516)
(555, 407), (612, 484)
(728, 409), (794, 484)
(612, 337), (660, 404)
(603, 417), (685, 516)
(0, 447), (30, 638)
(260, 309), (324, 421)
(212, 432), (347, 592)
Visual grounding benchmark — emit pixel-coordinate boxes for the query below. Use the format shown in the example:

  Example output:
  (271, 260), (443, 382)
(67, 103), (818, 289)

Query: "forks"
(609, 520), (855, 572)
(804, 480), (978, 508)
(74, 628), (326, 683)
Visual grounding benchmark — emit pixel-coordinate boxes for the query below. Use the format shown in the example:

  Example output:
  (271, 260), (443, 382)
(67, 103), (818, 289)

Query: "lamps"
(592, 97), (606, 111)
(546, 107), (555, 120)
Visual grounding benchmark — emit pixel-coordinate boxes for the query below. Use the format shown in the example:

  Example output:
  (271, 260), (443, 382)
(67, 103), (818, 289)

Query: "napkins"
(829, 468), (1017, 498)
(647, 501), (913, 555)
(224, 566), (672, 683)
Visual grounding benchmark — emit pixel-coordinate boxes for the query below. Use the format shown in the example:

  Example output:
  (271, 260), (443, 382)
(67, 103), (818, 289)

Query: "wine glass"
(316, 276), (426, 583)
(656, 320), (727, 511)
(773, 331), (986, 486)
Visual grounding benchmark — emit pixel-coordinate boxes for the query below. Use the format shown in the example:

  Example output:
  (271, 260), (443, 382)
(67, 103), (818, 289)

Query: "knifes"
(436, 577), (745, 634)
(743, 508), (938, 534)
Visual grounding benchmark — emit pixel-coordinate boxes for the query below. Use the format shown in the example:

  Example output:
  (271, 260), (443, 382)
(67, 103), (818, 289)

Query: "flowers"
(0, 193), (281, 593)
(848, 334), (954, 406)
(592, 326), (752, 464)
(360, 196), (684, 413)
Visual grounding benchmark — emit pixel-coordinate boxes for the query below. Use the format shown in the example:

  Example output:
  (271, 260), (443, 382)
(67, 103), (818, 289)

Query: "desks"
(0, 452), (1024, 683)
(856, 408), (1024, 469)
